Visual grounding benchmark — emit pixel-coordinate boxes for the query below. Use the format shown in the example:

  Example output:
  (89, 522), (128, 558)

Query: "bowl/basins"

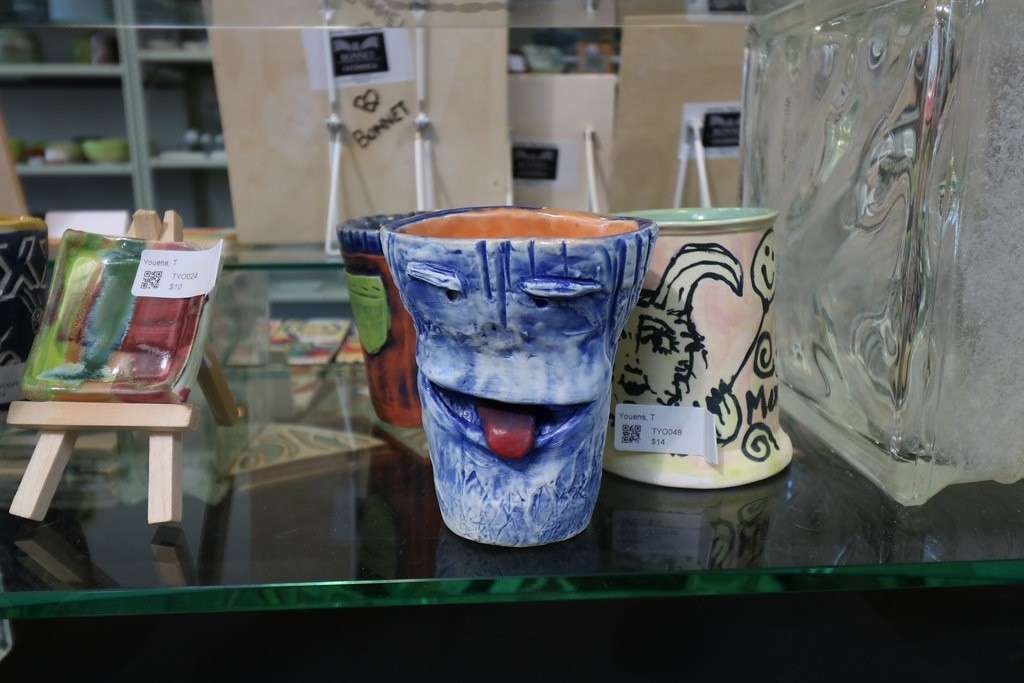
(10, 140), (131, 161)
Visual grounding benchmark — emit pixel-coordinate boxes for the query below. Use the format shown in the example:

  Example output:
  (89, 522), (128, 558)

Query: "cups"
(377, 204), (659, 547)
(337, 211), (432, 428)
(600, 209), (793, 488)
(0, 217), (54, 411)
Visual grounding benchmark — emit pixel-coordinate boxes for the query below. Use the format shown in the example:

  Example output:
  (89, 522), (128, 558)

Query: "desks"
(0, 258), (1024, 621)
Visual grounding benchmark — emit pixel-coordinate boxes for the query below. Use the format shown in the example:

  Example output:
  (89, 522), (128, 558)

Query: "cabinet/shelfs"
(0, 0), (237, 232)
